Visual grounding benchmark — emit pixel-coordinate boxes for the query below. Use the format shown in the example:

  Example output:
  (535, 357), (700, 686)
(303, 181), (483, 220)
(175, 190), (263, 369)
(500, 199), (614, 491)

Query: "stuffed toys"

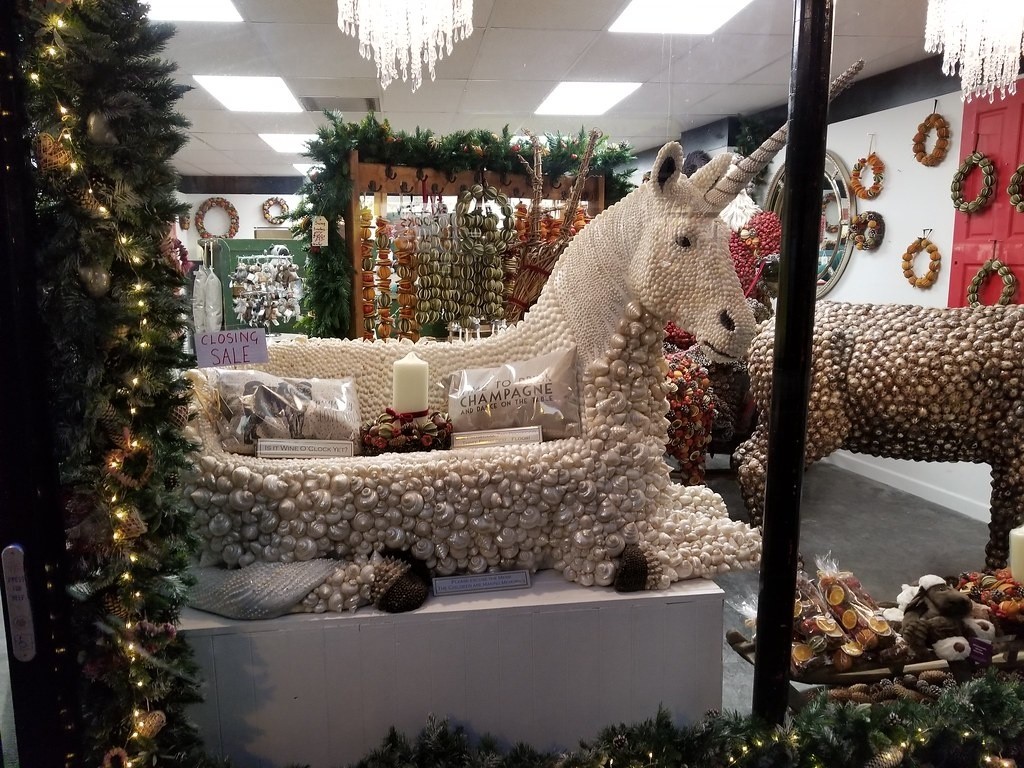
(880, 574), (995, 660)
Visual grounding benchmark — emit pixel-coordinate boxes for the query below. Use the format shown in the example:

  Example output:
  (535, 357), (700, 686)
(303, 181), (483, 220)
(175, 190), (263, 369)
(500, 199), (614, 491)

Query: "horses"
(727, 295), (1024, 581)
(159, 138), (757, 625)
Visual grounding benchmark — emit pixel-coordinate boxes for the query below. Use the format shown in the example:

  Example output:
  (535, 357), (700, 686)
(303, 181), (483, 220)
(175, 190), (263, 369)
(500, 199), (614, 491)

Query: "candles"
(394, 346), (431, 414)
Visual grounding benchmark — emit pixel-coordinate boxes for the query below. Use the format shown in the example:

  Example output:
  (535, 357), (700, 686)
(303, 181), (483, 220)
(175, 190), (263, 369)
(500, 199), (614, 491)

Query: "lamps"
(923, 0), (1024, 104)
(337, 0), (474, 94)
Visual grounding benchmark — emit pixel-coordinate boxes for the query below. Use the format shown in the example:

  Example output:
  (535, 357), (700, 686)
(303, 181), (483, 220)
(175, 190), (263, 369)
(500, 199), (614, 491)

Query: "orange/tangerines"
(794, 584), (892, 662)
